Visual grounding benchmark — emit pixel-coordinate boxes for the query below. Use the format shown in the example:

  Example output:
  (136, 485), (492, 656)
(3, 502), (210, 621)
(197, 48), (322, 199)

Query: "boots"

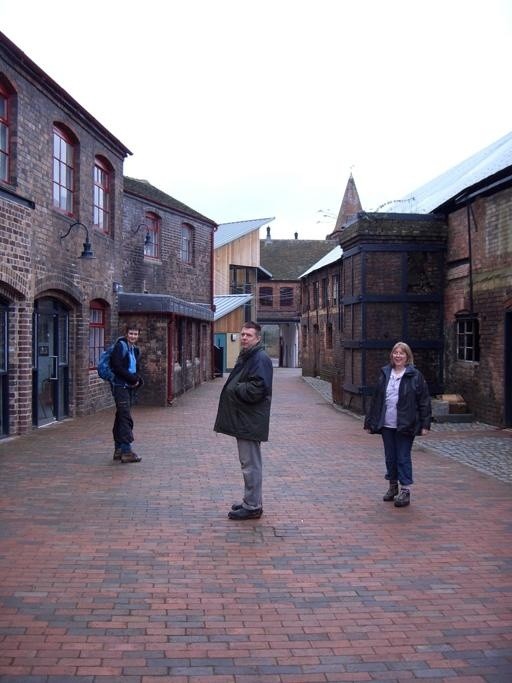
(394, 488), (410, 507)
(383, 480), (398, 501)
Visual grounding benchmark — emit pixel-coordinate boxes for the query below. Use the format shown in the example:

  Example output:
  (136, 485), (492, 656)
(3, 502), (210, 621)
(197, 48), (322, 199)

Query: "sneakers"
(113, 450), (142, 462)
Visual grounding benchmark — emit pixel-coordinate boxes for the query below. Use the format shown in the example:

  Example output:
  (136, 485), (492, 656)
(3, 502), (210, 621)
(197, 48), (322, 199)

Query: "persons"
(108, 324), (143, 463)
(214, 322), (273, 519)
(364, 342), (431, 507)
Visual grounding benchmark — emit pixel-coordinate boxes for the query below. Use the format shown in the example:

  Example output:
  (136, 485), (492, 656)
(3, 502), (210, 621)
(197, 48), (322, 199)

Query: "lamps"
(61, 222), (99, 260)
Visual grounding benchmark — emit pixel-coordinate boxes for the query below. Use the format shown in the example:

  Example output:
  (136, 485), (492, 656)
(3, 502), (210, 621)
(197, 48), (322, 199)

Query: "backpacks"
(97, 340), (128, 381)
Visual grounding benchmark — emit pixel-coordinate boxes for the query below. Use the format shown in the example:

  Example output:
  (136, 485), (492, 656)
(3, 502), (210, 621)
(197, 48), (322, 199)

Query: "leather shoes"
(228, 503), (262, 520)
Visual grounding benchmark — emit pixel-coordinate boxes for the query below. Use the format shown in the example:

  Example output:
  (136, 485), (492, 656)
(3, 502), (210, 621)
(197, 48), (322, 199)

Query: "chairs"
(38, 379), (53, 419)
